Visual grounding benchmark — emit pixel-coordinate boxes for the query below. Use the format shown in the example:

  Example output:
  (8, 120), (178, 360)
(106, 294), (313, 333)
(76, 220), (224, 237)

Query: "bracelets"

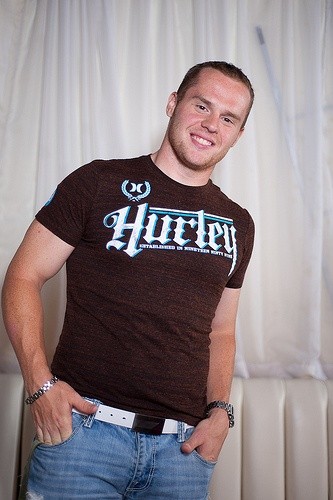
(23, 376), (59, 405)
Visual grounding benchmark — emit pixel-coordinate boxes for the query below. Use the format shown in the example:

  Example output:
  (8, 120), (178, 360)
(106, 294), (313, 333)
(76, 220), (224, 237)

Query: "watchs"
(203, 401), (235, 428)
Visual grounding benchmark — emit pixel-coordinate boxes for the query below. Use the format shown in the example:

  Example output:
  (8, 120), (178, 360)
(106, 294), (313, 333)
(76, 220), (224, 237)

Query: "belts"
(72, 399), (194, 435)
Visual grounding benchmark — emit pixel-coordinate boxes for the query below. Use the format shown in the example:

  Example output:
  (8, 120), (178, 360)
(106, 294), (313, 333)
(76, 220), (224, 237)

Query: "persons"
(0, 61), (256, 500)
